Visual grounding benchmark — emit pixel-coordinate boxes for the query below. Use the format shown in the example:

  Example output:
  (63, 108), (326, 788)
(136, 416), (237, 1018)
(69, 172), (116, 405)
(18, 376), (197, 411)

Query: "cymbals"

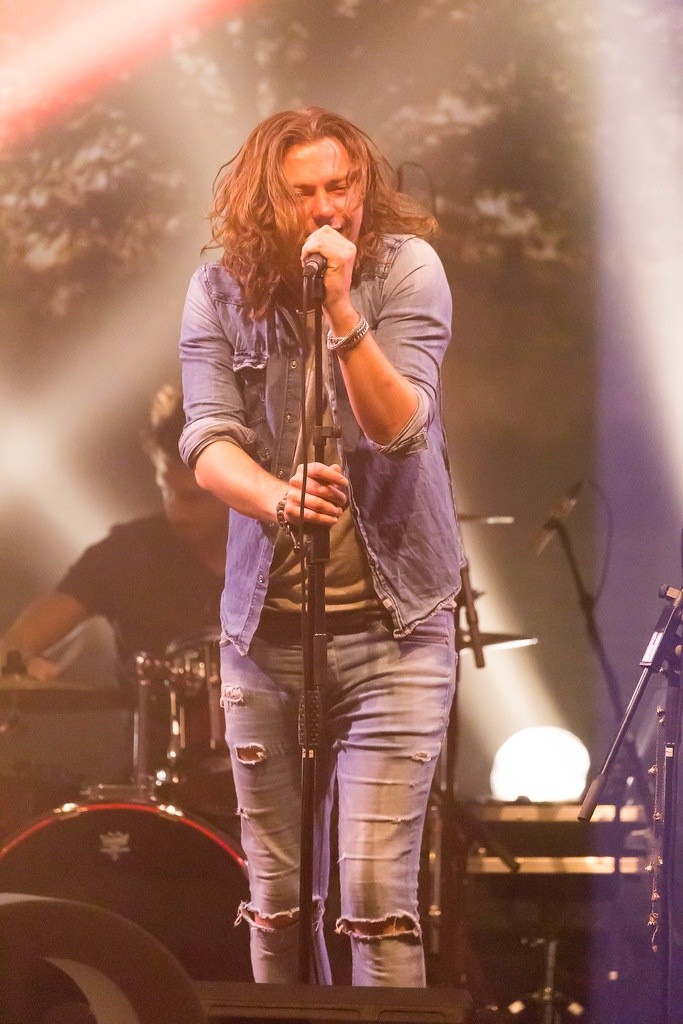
(453, 632), (539, 656)
(456, 511), (516, 527)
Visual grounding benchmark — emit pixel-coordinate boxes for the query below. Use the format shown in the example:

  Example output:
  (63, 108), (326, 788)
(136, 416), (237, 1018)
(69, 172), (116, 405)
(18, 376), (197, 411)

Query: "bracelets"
(324, 313), (369, 350)
(277, 490), (295, 532)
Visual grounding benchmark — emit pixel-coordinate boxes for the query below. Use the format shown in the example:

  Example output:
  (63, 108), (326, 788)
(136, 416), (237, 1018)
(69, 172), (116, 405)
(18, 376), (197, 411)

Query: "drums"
(141, 626), (239, 818)
(1, 783), (254, 981)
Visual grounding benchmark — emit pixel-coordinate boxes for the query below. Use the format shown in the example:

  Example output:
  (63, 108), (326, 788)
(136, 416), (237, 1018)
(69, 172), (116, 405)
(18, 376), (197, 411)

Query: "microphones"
(301, 252), (325, 278)
(527, 480), (586, 556)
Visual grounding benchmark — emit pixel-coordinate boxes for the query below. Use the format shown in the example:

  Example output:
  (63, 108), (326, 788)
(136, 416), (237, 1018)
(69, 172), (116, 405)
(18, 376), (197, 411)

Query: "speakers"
(193, 983), (486, 1024)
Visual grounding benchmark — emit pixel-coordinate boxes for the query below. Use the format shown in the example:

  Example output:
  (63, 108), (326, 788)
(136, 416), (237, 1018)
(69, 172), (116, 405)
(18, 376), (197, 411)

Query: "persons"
(176, 105), (470, 987)
(0, 381), (228, 680)
